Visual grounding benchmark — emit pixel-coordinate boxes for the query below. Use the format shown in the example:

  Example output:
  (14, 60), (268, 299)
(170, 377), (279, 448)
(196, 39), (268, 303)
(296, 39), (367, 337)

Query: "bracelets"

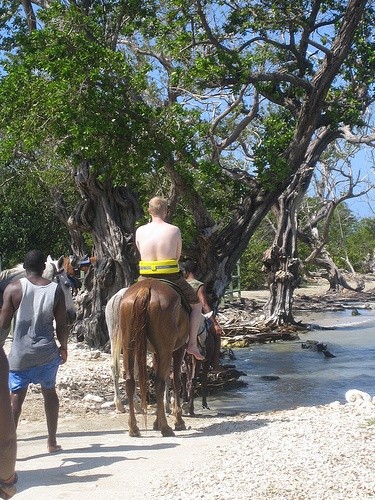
(0, 474), (15, 483)
(0, 474), (18, 488)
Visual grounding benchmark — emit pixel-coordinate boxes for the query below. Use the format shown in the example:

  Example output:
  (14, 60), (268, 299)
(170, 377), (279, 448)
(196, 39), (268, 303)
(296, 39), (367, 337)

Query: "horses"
(103, 278), (221, 439)
(1, 253), (78, 325)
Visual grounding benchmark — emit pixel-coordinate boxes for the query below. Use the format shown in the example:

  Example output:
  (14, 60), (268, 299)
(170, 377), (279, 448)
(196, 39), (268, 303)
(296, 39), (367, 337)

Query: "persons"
(136, 197), (205, 360)
(0, 249), (67, 452)
(180, 260), (228, 373)
(0, 345), (18, 500)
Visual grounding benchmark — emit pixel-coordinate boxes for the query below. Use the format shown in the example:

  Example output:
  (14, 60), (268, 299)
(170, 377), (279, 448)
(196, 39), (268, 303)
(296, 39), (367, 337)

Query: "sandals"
(47, 444), (62, 455)
